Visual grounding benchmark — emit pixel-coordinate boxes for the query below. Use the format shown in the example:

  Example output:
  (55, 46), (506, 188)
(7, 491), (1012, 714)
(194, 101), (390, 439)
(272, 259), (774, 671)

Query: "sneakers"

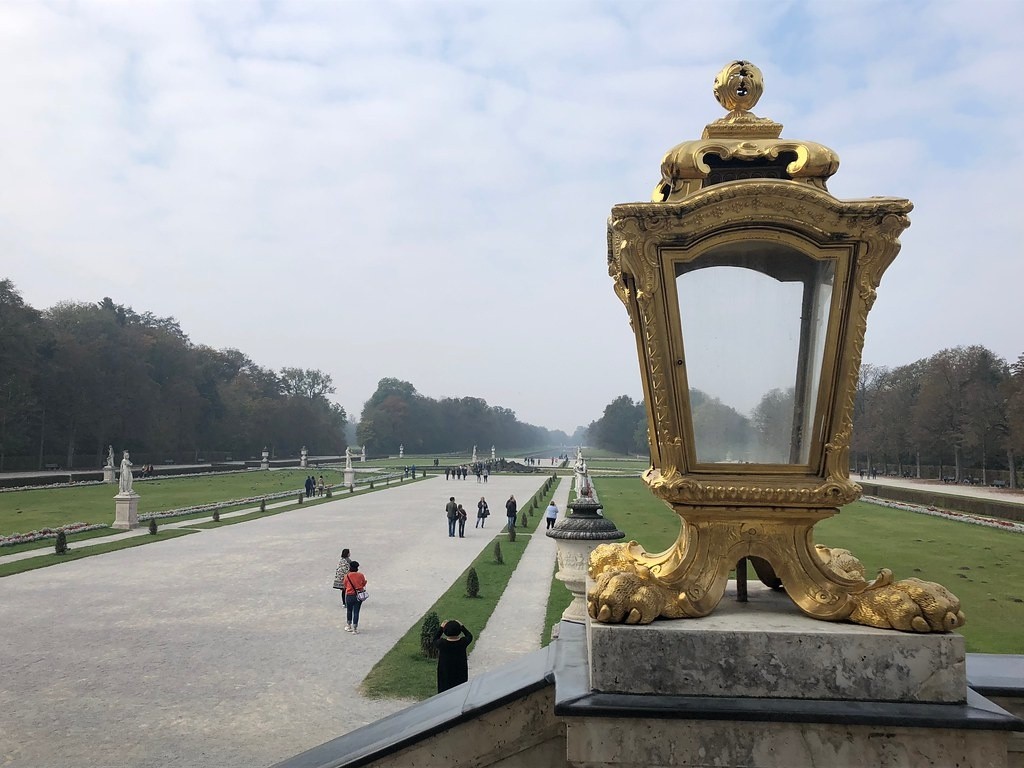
(352, 630), (360, 635)
(345, 626), (352, 632)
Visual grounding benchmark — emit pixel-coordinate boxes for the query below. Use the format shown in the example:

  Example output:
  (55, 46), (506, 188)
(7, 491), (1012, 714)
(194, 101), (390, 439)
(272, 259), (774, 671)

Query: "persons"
(119, 450), (133, 492)
(434, 458), (439, 466)
(551, 454), (568, 465)
(432, 620), (473, 694)
(574, 451), (588, 498)
(362, 444), (366, 455)
(346, 446), (354, 469)
(446, 497), (467, 538)
(141, 461), (153, 479)
(476, 497), (488, 528)
(547, 501), (558, 530)
(305, 475), (324, 498)
(859, 467), (974, 484)
(333, 549), (367, 634)
(506, 494), (518, 530)
(404, 464), (415, 478)
(525, 456), (540, 466)
(107, 445), (114, 466)
(445, 464), (492, 484)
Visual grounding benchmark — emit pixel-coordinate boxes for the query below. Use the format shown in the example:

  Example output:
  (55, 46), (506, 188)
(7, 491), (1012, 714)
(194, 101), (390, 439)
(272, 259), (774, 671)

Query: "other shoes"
(449, 535), (451, 537)
(452, 535), (455, 537)
(342, 604), (347, 608)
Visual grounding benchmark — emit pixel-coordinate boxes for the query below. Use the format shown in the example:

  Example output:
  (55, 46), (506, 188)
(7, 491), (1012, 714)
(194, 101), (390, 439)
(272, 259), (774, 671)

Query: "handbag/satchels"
(463, 516), (466, 520)
(356, 590), (369, 601)
(485, 507), (490, 515)
(318, 486), (323, 490)
(313, 484), (316, 489)
